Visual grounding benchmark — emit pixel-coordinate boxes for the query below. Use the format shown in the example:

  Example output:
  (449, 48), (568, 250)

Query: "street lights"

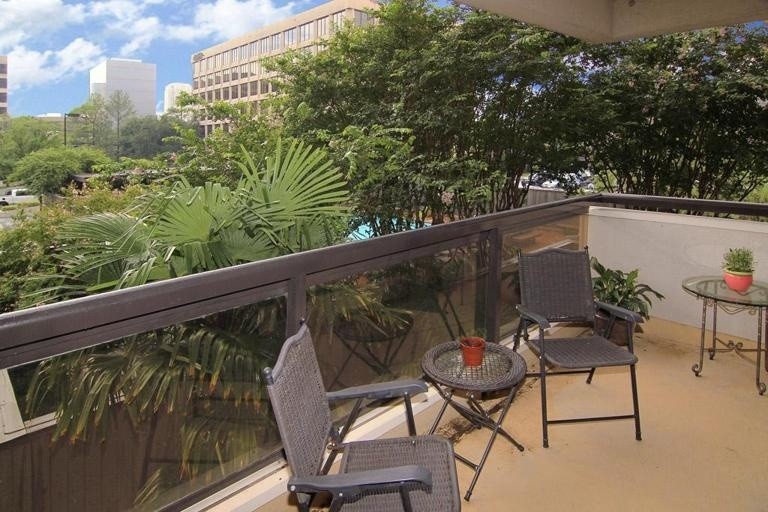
(64, 113), (80, 146)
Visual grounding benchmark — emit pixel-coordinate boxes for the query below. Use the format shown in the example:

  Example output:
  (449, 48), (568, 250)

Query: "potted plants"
(721, 247), (754, 293)
(589, 256), (666, 346)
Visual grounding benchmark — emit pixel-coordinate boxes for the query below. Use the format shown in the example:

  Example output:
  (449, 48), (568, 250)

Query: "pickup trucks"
(0, 188), (40, 206)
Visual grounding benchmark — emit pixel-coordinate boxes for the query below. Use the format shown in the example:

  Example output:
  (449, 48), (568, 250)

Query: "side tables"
(681, 276), (768, 396)
(327, 311), (414, 393)
(421, 340), (527, 501)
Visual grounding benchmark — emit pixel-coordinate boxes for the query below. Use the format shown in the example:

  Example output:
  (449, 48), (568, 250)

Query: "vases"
(459, 337), (486, 367)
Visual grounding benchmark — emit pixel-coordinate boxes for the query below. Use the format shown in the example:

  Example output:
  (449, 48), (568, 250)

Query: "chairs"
(512, 244), (644, 449)
(260, 316), (461, 511)
